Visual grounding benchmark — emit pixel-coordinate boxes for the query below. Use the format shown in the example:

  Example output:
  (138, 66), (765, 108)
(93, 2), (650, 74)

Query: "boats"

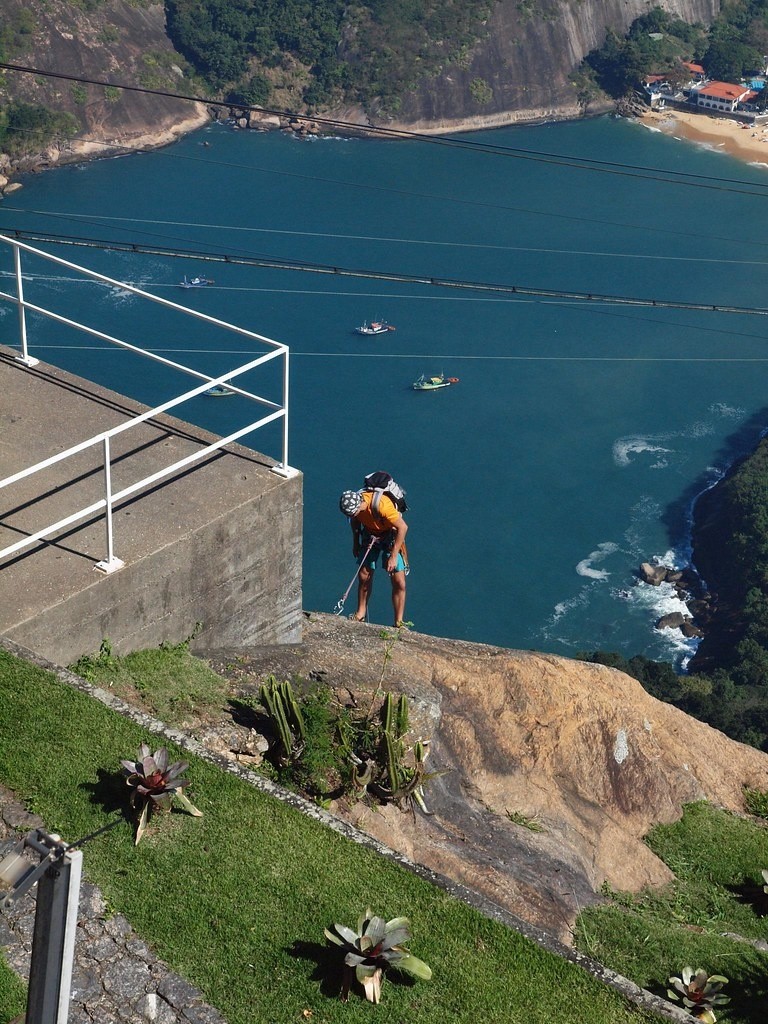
(414, 370), (460, 391)
(179, 272), (215, 290)
(202, 368), (236, 396)
(356, 315), (397, 335)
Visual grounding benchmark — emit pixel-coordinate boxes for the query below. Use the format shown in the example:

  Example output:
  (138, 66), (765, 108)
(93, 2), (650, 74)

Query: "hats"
(340, 490), (360, 516)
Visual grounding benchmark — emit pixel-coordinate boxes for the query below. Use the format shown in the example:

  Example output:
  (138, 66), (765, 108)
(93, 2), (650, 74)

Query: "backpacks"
(356, 471), (409, 519)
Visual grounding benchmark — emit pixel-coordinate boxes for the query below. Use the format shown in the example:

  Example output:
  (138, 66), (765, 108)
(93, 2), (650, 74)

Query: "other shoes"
(355, 614), (365, 622)
(393, 622), (407, 629)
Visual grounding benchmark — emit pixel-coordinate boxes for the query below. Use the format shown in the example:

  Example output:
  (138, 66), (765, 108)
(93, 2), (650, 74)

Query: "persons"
(340, 489), (409, 628)
